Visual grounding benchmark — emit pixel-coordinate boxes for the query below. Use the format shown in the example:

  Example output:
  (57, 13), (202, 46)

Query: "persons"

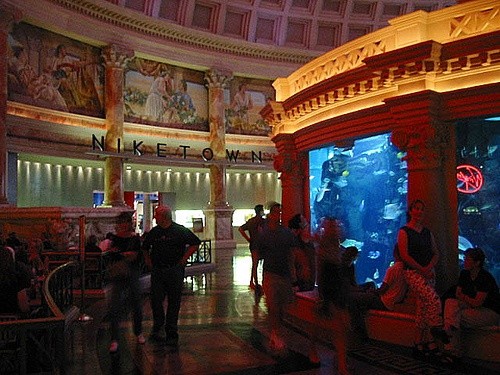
(0, 232), (114, 322)
(256, 201), (352, 375)
(340, 216), (408, 311)
(104, 211), (153, 353)
(142, 207), (202, 351)
(238, 205), (266, 288)
(398, 199), (443, 358)
(432, 246), (500, 365)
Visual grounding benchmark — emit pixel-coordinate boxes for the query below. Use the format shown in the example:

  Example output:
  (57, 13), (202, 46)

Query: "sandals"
(430, 328), (451, 343)
(440, 352), (460, 364)
(414, 341), (442, 355)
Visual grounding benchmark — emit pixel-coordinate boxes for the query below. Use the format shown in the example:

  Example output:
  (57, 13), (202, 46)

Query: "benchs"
(286, 291), (500, 362)
(40, 252), (102, 289)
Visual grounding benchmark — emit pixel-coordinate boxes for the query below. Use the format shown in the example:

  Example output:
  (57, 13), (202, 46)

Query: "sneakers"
(110, 343), (117, 352)
(138, 335), (145, 343)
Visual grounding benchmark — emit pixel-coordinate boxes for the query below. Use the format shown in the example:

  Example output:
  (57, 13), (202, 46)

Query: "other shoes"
(269, 337), (286, 351)
(150, 329), (179, 341)
(309, 349), (321, 361)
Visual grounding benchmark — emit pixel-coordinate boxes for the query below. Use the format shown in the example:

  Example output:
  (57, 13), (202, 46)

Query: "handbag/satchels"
(347, 300), (368, 329)
